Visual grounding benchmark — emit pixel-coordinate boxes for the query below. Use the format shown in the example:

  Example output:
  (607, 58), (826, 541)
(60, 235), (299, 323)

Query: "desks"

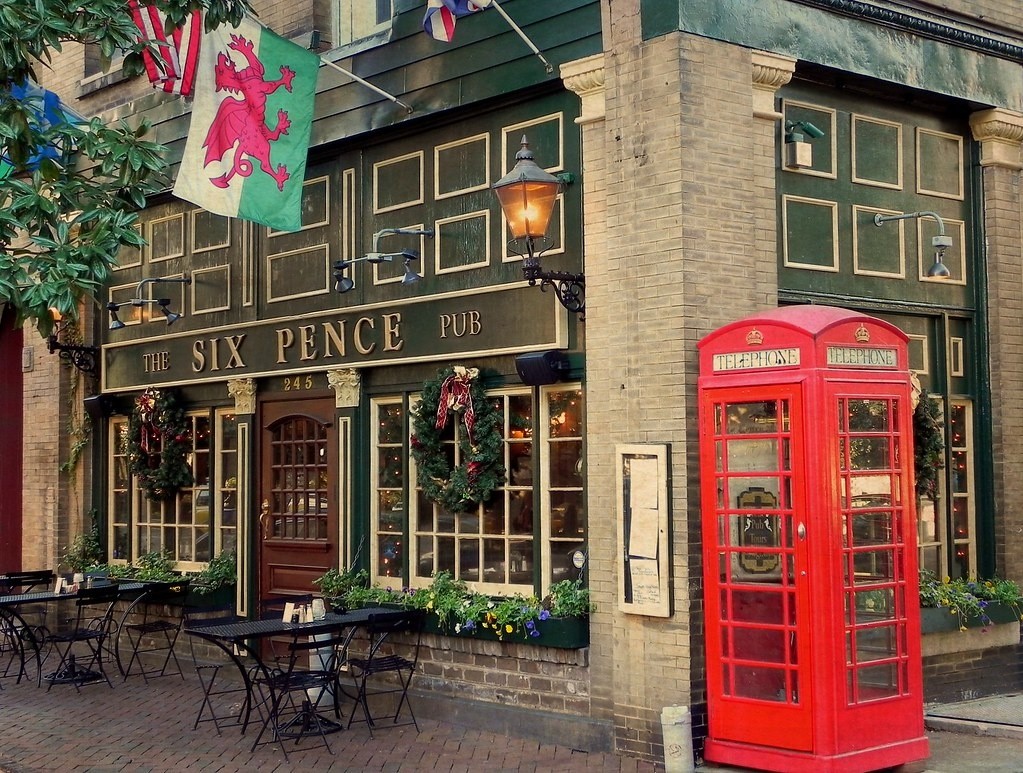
(184, 606), (405, 743)
(0, 580), (150, 682)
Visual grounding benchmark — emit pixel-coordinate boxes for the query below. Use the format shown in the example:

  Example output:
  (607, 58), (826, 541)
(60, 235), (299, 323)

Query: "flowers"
(849, 574), (1022, 633)
(321, 568), (597, 638)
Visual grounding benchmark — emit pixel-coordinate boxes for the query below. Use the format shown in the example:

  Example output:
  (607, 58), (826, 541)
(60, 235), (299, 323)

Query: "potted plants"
(59, 506), (238, 610)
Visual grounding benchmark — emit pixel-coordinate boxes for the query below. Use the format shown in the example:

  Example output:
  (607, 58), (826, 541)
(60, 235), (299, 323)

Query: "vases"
(844, 597), (1022, 636)
(331, 595), (591, 650)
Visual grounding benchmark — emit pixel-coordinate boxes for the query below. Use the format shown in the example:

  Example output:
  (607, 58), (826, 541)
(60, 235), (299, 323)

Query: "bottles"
(522, 556), (527, 572)
(299, 604), (314, 623)
(87, 575), (93, 589)
(62, 578), (67, 588)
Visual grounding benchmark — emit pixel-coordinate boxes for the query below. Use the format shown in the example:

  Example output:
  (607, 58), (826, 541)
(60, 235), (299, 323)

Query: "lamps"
(321, 223), (437, 291)
(41, 304), (101, 370)
(491, 130), (586, 324)
(872, 209), (954, 281)
(105, 274), (189, 327)
(784, 120), (826, 142)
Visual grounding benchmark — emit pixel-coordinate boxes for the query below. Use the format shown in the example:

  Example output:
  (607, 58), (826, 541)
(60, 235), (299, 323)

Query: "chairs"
(183, 604), (264, 737)
(44, 584), (119, 695)
(0, 570), (57, 683)
(121, 580), (190, 683)
(260, 593), (348, 727)
(333, 612), (422, 739)
(250, 624), (345, 764)
